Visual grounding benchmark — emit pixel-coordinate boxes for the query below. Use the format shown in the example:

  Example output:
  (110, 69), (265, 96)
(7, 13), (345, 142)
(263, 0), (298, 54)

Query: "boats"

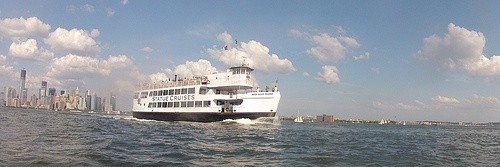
(132, 41), (281, 121)
